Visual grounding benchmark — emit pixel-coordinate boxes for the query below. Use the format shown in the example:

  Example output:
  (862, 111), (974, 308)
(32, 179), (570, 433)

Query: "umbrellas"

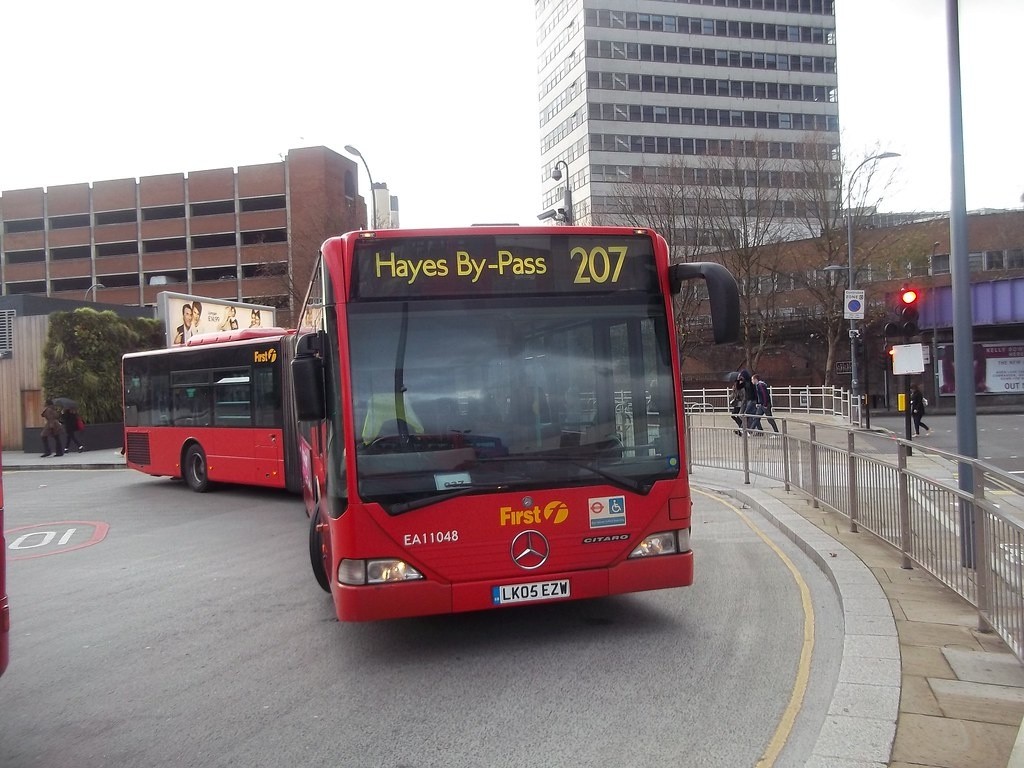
(53, 397), (80, 413)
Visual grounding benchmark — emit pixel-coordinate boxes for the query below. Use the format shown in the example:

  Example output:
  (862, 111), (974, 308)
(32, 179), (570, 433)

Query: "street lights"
(551, 160), (573, 226)
(931, 241), (941, 408)
(822, 152), (903, 427)
(344, 145), (377, 230)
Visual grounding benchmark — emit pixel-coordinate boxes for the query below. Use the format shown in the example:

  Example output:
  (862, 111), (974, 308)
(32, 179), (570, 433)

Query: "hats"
(910, 383), (917, 390)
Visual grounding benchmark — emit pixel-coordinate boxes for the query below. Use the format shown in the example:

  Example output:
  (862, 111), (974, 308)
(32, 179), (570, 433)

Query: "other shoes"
(54, 454), (63, 457)
(78, 446), (84, 453)
(41, 454), (51, 458)
(926, 428), (932, 436)
(911, 434), (919, 437)
(64, 449), (68, 452)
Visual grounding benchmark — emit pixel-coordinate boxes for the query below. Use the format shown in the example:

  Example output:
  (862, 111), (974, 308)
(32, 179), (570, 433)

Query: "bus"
(119, 220), (742, 625)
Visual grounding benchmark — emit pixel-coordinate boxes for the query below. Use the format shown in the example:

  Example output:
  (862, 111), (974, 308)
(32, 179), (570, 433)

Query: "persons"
(910, 384), (931, 437)
(40, 400), (64, 457)
(728, 370), (780, 439)
(939, 344), (988, 394)
(173, 301), (202, 344)
(216, 306), (239, 332)
(248, 310), (260, 328)
(59, 408), (84, 453)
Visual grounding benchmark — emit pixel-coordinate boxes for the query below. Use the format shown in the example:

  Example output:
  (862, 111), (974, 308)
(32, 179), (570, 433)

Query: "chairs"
(361, 392), (424, 445)
(512, 385), (551, 423)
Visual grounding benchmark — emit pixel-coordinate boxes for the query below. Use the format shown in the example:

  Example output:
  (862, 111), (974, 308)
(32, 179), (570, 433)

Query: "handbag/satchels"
(74, 416), (85, 431)
(922, 398), (928, 407)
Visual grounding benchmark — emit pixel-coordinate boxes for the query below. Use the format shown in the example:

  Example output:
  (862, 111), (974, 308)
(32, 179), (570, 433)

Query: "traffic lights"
(883, 321), (899, 337)
(897, 288), (920, 337)
